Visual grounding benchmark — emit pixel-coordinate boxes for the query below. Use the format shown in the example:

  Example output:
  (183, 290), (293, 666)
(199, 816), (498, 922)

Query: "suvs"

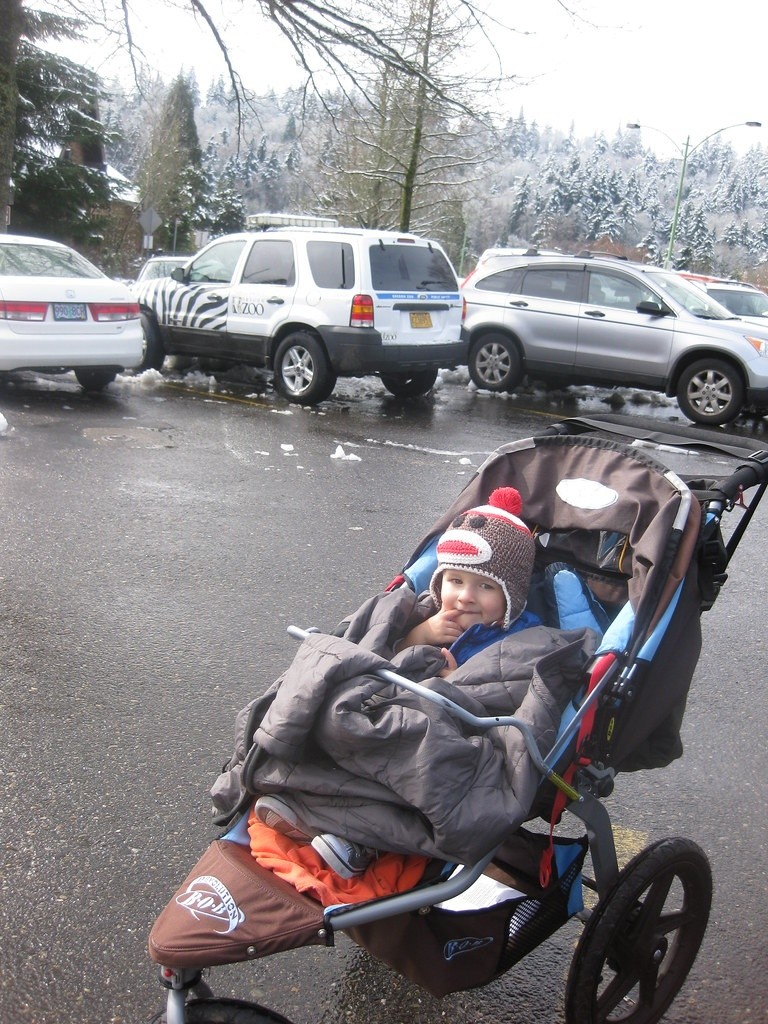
(460, 250), (768, 425)
(647, 279), (768, 324)
(135, 248), (223, 284)
(118, 227), (466, 406)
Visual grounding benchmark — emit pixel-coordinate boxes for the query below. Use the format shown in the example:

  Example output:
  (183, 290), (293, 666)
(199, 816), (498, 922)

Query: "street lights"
(626, 121), (761, 270)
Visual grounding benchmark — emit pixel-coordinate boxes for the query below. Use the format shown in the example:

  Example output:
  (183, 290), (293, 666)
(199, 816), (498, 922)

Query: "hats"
(429, 488), (535, 630)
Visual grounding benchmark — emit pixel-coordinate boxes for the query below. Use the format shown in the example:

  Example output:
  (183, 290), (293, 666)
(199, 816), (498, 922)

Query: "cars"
(0, 233), (148, 391)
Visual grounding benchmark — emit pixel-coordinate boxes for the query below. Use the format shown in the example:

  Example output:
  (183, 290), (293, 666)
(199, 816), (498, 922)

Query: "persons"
(254, 487), (542, 880)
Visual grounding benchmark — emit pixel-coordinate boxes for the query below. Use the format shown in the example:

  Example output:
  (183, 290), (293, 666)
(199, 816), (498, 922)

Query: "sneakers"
(254, 792), (321, 844)
(312, 835), (380, 878)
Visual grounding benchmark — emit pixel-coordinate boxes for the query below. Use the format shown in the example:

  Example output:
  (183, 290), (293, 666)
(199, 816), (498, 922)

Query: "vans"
(474, 248), (654, 303)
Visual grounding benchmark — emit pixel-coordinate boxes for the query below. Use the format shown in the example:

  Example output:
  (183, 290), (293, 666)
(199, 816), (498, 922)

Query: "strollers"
(149, 415), (768, 1024)
